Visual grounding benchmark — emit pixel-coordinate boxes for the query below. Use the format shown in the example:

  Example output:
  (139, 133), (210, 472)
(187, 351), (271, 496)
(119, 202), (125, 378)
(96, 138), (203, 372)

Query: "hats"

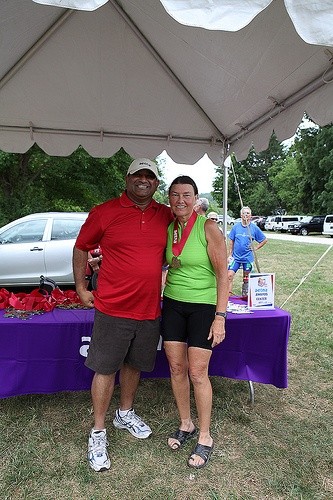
(207, 212), (219, 220)
(126, 158), (162, 181)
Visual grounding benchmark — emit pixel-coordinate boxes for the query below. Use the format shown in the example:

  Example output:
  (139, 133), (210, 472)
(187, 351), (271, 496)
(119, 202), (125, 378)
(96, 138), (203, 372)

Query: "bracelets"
(97, 254), (102, 261)
(215, 311), (227, 318)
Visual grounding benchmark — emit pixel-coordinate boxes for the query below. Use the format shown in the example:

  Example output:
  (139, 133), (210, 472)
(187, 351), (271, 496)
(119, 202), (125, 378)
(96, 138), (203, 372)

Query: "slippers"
(187, 439), (215, 469)
(167, 428), (197, 452)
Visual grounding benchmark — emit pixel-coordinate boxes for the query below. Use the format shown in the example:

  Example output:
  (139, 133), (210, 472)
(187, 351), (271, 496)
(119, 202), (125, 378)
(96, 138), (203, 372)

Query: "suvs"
(287, 215), (325, 236)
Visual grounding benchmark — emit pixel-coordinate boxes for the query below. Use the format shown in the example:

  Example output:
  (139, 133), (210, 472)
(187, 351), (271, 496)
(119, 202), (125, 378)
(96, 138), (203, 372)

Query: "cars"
(216, 214), (277, 233)
(0, 212), (89, 288)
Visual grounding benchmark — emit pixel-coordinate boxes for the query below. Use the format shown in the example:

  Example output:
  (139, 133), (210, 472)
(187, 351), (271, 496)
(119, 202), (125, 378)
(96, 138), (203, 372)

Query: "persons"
(72, 158), (175, 473)
(160, 176), (229, 469)
(85, 197), (224, 298)
(228, 206), (268, 297)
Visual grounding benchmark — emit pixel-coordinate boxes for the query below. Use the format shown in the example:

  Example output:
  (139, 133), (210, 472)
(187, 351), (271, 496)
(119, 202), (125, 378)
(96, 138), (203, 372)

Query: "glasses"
(210, 218), (218, 221)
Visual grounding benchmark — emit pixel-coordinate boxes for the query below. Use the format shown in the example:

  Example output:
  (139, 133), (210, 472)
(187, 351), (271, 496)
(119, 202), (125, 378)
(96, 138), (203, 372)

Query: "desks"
(0, 295), (291, 405)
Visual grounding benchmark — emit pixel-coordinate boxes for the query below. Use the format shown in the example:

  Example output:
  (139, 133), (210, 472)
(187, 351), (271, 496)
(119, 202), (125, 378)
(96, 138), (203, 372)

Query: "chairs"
(52, 225), (68, 240)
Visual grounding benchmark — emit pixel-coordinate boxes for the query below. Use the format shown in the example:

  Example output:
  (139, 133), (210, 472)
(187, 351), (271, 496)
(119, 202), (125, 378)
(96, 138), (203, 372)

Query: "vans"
(274, 216), (307, 233)
(322, 215), (333, 238)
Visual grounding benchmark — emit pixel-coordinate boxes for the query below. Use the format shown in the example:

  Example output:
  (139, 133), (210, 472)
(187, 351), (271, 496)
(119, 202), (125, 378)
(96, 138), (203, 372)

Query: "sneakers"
(88, 428), (111, 471)
(113, 409), (153, 439)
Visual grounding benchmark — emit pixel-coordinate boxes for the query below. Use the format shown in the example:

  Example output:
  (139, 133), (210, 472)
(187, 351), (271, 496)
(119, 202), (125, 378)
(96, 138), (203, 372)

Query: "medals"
(171, 256), (181, 269)
